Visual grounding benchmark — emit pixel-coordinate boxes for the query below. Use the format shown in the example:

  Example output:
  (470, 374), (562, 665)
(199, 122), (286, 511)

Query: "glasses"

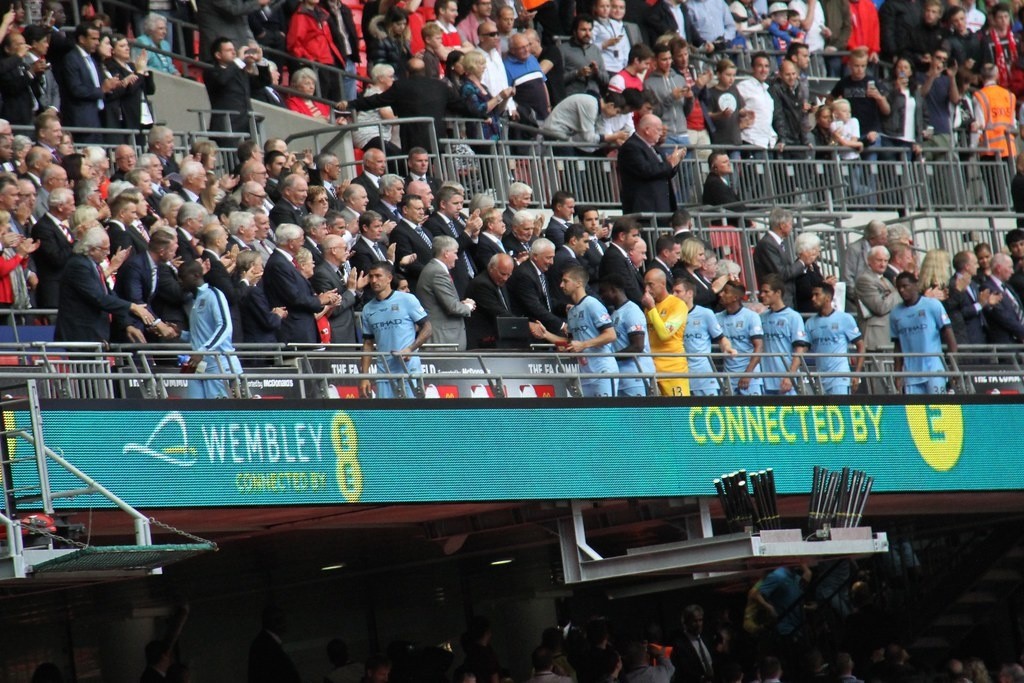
(98, 246), (111, 251)
(482, 32), (499, 37)
(344, 247), (348, 252)
(307, 261), (315, 264)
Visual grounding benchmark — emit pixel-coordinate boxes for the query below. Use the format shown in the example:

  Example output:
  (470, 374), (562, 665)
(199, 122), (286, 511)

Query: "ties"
(52, 151), (300, 322)
(329, 178), (674, 313)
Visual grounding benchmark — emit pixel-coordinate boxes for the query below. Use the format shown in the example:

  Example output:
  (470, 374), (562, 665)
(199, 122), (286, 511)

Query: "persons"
(0, 0), (1024, 399)
(28, 538), (1024, 683)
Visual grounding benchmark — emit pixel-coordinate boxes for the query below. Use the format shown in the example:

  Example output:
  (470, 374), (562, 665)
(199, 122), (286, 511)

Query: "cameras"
(45, 62), (52, 70)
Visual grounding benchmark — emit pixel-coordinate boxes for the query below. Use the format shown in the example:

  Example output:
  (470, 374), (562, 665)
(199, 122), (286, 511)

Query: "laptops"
(497, 316), (530, 348)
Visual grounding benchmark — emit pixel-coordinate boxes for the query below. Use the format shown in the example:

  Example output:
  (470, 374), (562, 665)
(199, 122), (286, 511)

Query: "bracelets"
(497, 94), (503, 102)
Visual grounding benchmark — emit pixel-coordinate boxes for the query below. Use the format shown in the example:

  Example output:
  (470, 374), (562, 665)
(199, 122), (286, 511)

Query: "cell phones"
(867, 81), (875, 90)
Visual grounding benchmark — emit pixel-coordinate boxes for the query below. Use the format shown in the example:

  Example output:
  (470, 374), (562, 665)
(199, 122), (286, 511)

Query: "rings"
(145, 316), (147, 318)
(677, 153), (678, 155)
